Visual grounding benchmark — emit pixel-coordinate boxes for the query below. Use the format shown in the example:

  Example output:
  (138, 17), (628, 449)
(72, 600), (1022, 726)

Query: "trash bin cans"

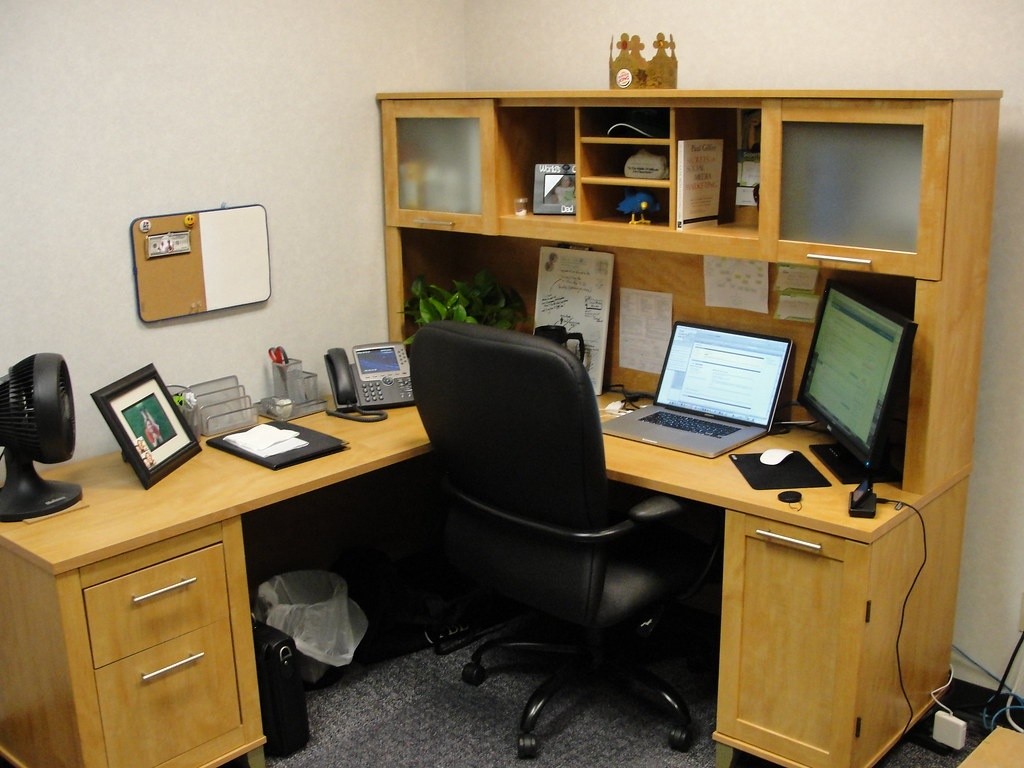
(259, 569), (347, 691)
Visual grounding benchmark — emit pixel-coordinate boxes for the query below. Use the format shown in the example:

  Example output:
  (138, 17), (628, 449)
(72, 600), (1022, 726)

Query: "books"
(676, 140), (723, 230)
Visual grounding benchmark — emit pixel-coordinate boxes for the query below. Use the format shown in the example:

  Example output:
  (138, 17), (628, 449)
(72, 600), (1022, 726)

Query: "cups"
(534, 325), (584, 366)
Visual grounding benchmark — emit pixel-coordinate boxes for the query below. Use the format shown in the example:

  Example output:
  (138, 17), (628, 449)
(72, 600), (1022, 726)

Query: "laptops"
(600, 321), (793, 457)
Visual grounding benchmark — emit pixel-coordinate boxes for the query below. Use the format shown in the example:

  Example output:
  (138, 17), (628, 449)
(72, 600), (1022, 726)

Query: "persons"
(554, 175), (575, 203)
(144, 452), (156, 468)
(140, 408), (165, 447)
(136, 440), (148, 454)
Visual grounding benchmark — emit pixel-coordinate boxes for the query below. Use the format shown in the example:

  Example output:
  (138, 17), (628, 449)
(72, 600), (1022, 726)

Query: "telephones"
(324, 341), (417, 413)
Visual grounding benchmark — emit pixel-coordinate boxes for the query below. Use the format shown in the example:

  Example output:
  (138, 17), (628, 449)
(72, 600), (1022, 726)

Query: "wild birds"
(616, 190), (661, 225)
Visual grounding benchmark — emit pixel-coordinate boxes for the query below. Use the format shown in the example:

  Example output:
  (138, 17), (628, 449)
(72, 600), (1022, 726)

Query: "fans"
(0, 352), (83, 522)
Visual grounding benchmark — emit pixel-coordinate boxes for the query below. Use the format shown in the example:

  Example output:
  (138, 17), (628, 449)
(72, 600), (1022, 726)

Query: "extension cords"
(906, 726), (954, 756)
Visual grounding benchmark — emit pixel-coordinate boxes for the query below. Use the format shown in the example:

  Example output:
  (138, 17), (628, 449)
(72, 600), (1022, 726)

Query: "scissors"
(269, 346), (288, 398)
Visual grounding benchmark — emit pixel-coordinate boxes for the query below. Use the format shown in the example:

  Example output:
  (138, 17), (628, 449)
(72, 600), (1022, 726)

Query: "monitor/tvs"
(796, 278), (920, 482)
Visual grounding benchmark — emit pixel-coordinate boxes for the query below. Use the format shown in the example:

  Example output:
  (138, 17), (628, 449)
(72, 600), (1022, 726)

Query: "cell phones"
(852, 477), (873, 508)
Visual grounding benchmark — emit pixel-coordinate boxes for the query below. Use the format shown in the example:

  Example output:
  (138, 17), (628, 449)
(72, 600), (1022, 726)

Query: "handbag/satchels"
(250, 613), (308, 753)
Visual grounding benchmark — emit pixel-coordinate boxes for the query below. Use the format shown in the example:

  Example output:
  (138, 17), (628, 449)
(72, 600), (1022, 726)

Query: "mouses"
(760, 449), (793, 466)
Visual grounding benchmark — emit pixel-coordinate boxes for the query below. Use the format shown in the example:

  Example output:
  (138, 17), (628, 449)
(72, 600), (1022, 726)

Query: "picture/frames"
(533, 163), (579, 214)
(90, 364), (203, 490)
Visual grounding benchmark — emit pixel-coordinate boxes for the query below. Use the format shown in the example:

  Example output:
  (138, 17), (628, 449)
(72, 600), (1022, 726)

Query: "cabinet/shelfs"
(374, 90), (1004, 503)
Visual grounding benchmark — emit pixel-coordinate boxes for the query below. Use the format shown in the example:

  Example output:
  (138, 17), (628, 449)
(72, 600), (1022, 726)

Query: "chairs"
(405, 319), (717, 760)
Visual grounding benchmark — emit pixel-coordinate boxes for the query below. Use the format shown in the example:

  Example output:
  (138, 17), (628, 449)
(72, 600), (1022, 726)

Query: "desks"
(1, 383), (969, 768)
